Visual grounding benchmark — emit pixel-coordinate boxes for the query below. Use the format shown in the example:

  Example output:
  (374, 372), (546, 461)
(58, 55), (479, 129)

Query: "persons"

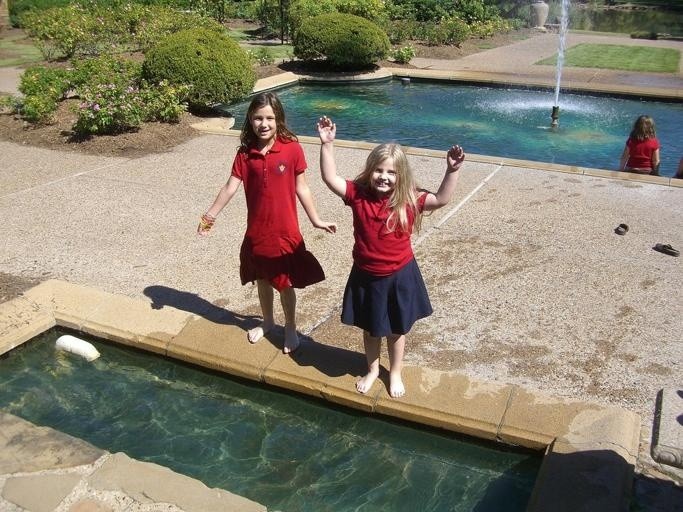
(620, 115), (660, 177)
(316, 115), (465, 398)
(672, 155), (683, 180)
(198, 92), (337, 354)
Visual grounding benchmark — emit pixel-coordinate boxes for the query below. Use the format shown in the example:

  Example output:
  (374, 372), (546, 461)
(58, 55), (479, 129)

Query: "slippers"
(655, 244), (680, 256)
(616, 224), (628, 235)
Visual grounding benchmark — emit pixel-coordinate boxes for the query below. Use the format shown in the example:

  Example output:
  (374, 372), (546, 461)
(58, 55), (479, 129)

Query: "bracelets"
(199, 213), (215, 232)
